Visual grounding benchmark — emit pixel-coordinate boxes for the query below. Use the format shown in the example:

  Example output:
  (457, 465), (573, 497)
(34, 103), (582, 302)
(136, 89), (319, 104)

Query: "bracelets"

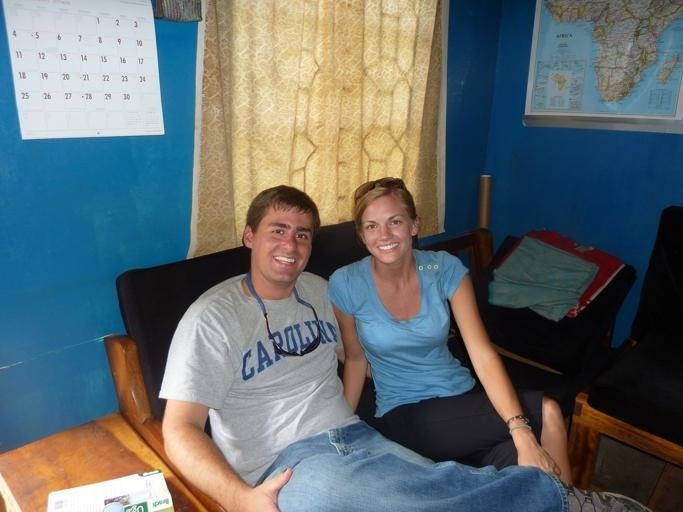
(509, 426), (534, 432)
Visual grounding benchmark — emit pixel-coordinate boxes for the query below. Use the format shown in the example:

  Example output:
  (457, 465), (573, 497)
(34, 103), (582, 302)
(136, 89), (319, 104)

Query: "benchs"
(103, 222), (636, 512)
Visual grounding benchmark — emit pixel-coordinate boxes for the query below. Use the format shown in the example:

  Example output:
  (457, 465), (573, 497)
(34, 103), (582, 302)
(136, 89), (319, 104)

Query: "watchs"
(505, 416), (530, 427)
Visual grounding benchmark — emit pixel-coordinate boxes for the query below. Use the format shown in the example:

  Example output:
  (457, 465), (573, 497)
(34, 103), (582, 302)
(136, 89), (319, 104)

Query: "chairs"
(567, 206), (682, 512)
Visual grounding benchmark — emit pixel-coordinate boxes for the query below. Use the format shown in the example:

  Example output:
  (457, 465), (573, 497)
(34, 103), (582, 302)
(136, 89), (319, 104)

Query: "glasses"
(264, 306), (321, 356)
(353, 177), (409, 207)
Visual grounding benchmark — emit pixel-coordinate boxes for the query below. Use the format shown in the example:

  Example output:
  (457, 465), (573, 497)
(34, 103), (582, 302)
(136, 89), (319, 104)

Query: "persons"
(327, 177), (574, 488)
(156, 184), (655, 512)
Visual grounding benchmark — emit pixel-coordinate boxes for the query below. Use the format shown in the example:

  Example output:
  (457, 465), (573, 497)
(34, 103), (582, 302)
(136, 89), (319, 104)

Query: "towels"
(484, 233), (598, 325)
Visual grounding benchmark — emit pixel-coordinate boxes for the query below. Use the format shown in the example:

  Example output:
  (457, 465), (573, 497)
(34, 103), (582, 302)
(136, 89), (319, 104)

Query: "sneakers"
(568, 488), (653, 512)
